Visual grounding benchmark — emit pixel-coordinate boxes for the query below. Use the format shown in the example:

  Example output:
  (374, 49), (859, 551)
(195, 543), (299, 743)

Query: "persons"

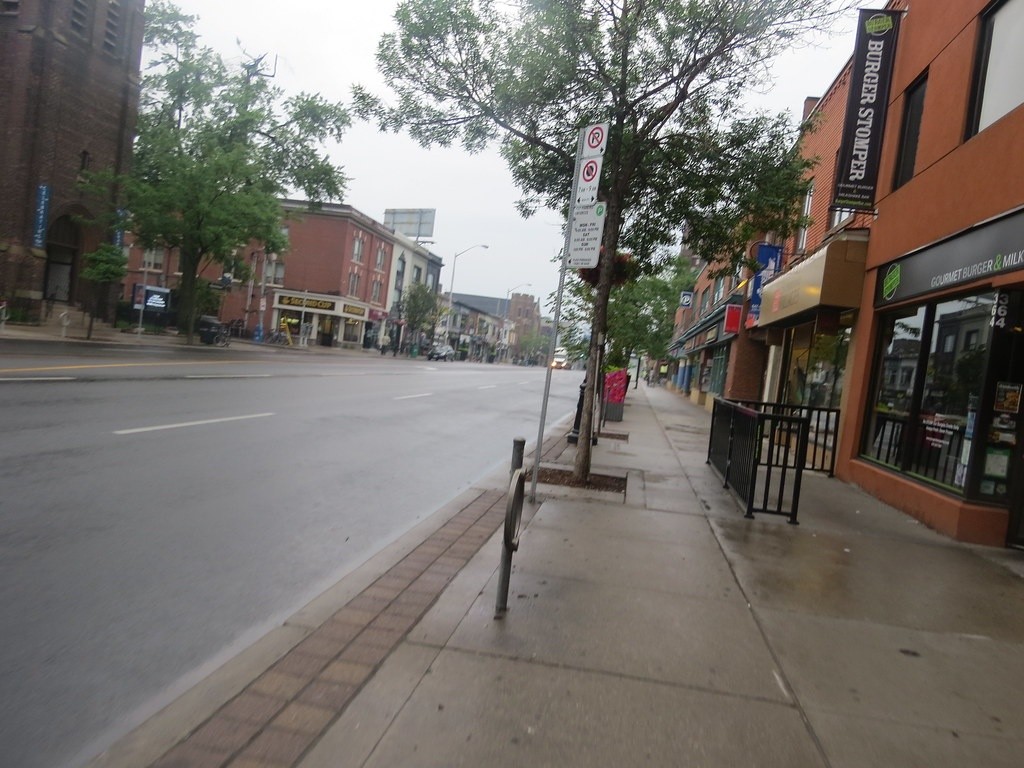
(380, 332), (391, 355)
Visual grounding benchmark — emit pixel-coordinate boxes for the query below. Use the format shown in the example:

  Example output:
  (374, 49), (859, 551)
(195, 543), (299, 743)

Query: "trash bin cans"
(198, 315), (222, 346)
(455, 351), (461, 361)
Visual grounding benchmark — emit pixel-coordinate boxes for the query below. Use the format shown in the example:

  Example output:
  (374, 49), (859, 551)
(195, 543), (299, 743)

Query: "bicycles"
(263, 329), (290, 346)
(213, 327), (233, 348)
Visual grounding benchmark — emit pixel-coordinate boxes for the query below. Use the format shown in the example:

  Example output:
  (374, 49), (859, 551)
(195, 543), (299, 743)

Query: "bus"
(552, 347), (571, 369)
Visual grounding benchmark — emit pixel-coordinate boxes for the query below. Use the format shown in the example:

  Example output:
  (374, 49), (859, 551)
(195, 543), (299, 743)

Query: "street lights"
(496, 282), (532, 366)
(443, 243), (488, 346)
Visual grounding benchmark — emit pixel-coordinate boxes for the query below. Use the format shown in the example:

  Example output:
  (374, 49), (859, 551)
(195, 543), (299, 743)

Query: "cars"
(429, 345), (456, 362)
